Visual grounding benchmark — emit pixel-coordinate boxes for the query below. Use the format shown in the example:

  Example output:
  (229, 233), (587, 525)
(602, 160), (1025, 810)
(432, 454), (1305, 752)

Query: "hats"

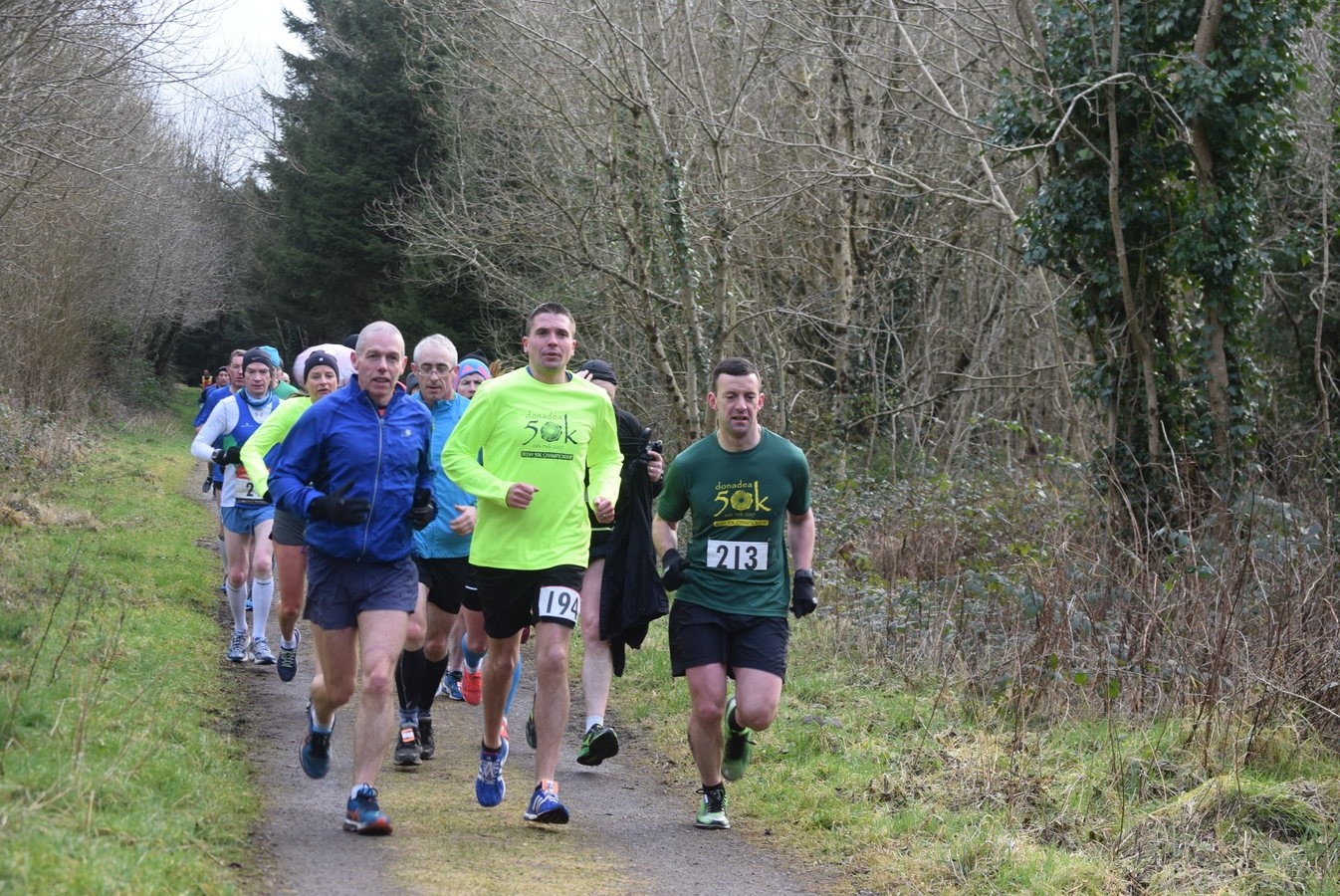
(452, 358), (491, 392)
(242, 343), (281, 379)
(578, 359), (617, 385)
(303, 349), (340, 385)
(459, 350), (489, 367)
(406, 372), (419, 395)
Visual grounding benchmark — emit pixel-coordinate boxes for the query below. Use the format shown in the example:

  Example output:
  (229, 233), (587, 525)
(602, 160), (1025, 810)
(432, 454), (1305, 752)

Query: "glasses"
(413, 362), (455, 377)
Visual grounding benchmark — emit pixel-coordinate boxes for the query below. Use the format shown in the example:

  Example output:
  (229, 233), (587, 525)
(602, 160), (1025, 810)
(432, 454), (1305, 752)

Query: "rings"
(603, 514), (606, 519)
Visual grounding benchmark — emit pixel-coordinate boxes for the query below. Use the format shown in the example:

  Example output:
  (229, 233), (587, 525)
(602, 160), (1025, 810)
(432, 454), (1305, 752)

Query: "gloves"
(790, 569), (817, 620)
(661, 549), (690, 592)
(211, 446), (243, 466)
(407, 488), (434, 531)
(310, 479), (371, 526)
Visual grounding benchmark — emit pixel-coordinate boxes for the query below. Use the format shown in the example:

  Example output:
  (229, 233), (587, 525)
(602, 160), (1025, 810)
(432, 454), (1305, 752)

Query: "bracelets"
(793, 568), (814, 579)
(659, 471), (664, 481)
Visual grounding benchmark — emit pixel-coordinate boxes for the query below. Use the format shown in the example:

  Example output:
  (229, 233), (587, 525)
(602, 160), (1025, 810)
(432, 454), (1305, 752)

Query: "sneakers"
(576, 723), (619, 766)
(525, 692), (538, 749)
(461, 657), (484, 706)
(522, 779), (569, 825)
(248, 636), (276, 664)
(202, 474), (253, 611)
(721, 696), (757, 781)
(299, 698), (336, 779)
(693, 788), (731, 830)
(393, 717), (436, 765)
(441, 666), (466, 700)
(343, 782), (393, 834)
(499, 716), (510, 741)
(224, 630), (252, 664)
(476, 735), (509, 807)
(276, 627), (301, 683)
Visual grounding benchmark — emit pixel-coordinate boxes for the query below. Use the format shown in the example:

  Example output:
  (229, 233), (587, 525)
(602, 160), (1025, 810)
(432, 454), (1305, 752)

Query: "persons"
(188, 340), (553, 768)
(522, 353), (668, 765)
(441, 300), (625, 836)
(649, 355), (817, 831)
(264, 318), (439, 838)
